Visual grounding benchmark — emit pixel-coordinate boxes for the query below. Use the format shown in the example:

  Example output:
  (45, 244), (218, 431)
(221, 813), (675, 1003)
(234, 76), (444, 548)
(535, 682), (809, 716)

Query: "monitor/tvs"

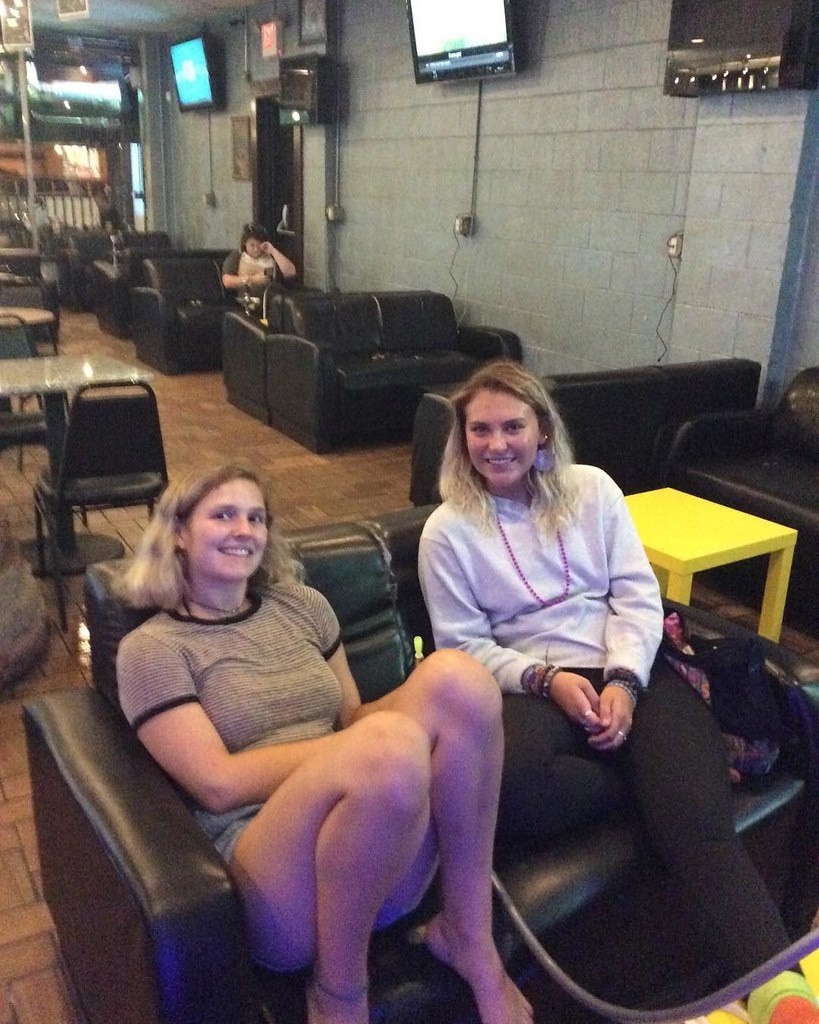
(405, 0), (517, 84)
(169, 31), (223, 114)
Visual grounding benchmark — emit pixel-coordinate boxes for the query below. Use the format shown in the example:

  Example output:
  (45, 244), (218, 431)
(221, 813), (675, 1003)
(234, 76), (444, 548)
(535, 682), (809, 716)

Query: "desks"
(625, 486), (798, 645)
(0, 307), (71, 413)
(0, 354), (155, 577)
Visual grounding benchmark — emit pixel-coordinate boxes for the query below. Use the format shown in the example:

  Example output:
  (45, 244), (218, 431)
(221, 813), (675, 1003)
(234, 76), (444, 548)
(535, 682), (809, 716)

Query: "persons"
(95, 190), (120, 234)
(418, 362), (819, 1024)
(222, 221), (297, 300)
(114, 455), (535, 1023)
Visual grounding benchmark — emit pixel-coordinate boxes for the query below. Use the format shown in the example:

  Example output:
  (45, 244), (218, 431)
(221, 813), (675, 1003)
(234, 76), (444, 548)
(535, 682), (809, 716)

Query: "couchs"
(24, 231), (819, 1024)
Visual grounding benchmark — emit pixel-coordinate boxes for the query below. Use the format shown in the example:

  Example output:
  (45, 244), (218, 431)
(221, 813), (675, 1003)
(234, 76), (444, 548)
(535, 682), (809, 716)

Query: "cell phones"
(264, 266), (274, 281)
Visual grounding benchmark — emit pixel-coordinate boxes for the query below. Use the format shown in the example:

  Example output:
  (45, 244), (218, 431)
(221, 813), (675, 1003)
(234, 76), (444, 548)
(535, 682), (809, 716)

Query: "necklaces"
(184, 596), (248, 614)
(495, 501), (570, 607)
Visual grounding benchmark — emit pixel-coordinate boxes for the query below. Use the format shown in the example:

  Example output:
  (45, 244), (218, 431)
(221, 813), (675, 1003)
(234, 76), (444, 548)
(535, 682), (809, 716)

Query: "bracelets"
(606, 670), (641, 708)
(522, 662), (561, 699)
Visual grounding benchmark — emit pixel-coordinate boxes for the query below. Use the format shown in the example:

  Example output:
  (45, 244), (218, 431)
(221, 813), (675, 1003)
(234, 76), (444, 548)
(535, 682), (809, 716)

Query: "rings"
(618, 731), (627, 740)
(585, 710), (592, 715)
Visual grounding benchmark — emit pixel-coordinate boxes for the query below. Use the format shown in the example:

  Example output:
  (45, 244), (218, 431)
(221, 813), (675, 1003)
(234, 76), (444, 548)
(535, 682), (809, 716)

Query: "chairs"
(0, 230), (45, 411)
(34, 382), (169, 634)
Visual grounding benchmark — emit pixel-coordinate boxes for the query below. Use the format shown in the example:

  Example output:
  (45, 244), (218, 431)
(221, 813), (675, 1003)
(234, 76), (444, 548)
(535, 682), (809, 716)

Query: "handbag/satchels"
(659, 630), (789, 799)
